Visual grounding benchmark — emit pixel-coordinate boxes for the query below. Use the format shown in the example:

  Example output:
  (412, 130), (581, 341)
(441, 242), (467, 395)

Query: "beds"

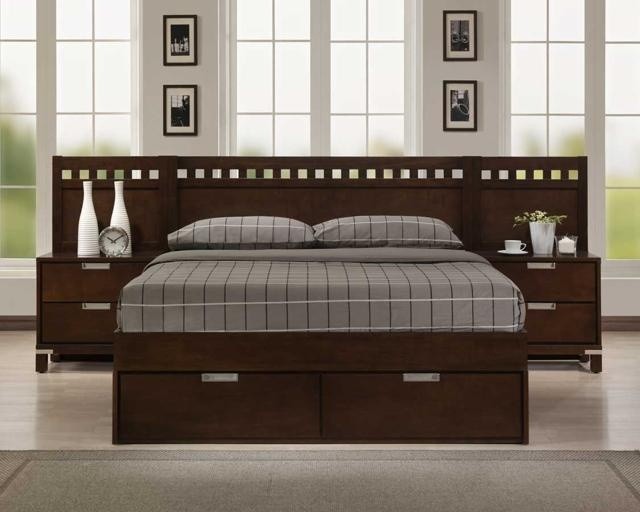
(109, 156), (525, 446)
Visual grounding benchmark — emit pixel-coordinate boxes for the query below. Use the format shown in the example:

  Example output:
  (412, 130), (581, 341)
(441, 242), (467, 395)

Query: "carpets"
(0, 448), (640, 512)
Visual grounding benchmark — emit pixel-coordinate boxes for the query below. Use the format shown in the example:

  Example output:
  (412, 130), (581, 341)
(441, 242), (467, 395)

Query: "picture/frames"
(443, 79), (478, 132)
(442, 9), (479, 62)
(161, 13), (199, 67)
(162, 84), (200, 138)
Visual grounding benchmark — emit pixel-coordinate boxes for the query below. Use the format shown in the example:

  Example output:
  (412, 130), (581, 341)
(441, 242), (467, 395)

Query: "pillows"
(313, 215), (466, 251)
(167, 216), (317, 250)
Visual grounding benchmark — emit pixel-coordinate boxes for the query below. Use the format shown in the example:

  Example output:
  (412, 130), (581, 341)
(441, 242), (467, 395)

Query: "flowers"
(512, 208), (565, 225)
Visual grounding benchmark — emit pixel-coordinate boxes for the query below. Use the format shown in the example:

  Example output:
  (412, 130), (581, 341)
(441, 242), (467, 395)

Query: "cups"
(504, 239), (526, 252)
(554, 236), (578, 254)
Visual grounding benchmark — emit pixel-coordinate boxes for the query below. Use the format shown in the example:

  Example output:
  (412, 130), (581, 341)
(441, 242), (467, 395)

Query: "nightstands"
(35, 252), (165, 371)
(479, 244), (602, 368)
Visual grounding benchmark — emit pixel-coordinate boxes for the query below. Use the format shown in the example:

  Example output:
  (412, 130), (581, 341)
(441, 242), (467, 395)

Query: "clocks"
(97, 223), (129, 256)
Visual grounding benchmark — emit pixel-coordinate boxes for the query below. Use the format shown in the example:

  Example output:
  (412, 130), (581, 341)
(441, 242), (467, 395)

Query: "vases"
(529, 222), (556, 255)
(77, 180), (101, 257)
(110, 180), (133, 257)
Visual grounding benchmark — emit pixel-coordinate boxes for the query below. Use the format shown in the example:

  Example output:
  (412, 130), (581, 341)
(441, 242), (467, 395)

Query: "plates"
(497, 251), (528, 255)
